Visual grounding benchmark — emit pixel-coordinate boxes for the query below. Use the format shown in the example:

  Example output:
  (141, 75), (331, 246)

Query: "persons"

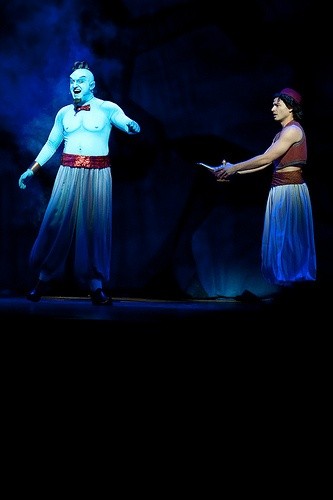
(19, 62), (140, 307)
(213, 89), (316, 300)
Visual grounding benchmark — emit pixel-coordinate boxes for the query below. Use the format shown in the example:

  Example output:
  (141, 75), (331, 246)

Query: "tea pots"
(198, 160), (229, 181)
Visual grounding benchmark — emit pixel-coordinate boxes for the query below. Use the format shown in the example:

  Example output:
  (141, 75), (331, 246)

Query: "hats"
(280, 86), (306, 107)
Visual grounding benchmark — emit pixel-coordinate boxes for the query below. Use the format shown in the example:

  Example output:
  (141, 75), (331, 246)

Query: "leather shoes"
(90, 286), (114, 305)
(29, 280), (45, 302)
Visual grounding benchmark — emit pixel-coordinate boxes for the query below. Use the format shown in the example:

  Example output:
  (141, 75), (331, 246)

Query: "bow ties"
(75, 104), (90, 112)
